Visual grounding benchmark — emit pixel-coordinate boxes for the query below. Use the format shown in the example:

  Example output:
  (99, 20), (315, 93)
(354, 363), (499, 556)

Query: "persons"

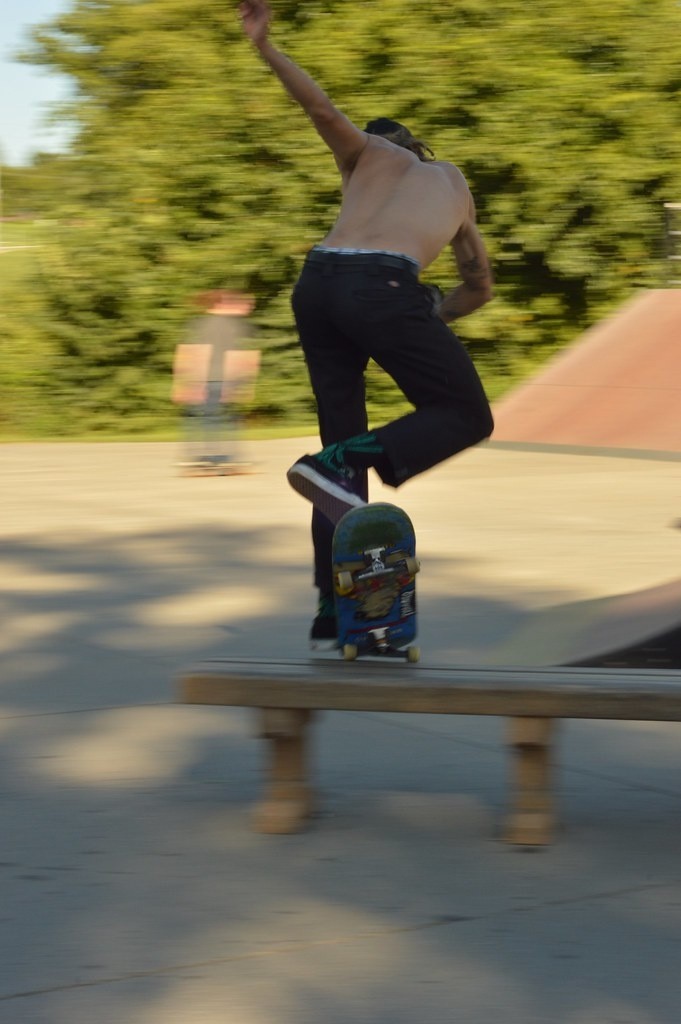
(235, 0), (494, 660)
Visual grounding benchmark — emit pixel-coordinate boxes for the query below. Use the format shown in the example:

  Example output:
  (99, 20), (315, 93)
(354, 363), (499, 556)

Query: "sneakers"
(287, 454), (369, 528)
(308, 614), (338, 654)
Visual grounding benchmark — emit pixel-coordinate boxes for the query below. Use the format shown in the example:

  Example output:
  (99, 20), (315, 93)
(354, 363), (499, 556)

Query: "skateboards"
(329, 502), (423, 664)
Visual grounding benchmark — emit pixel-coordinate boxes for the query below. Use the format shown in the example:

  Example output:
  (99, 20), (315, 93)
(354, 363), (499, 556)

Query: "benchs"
(179, 656), (681, 849)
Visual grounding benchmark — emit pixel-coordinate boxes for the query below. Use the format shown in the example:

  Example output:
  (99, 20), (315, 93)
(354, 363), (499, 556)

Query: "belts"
(304, 251), (419, 277)
